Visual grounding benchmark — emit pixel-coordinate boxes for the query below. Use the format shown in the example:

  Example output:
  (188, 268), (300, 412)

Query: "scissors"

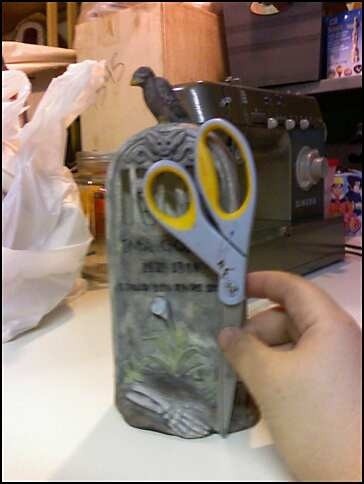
(142, 117), (259, 436)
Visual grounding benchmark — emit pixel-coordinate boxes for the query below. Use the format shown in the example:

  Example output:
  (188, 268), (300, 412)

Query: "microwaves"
(170, 80), (346, 276)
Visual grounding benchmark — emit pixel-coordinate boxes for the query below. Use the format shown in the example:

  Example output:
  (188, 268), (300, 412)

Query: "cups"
(72, 150), (137, 288)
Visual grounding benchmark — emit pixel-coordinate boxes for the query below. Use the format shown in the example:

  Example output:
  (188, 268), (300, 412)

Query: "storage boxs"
(70, 1), (224, 149)
(220, 2), (322, 88)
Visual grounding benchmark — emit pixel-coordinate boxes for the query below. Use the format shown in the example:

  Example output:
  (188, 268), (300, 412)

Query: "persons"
(344, 176), (363, 216)
(217, 270), (362, 482)
(330, 177), (348, 203)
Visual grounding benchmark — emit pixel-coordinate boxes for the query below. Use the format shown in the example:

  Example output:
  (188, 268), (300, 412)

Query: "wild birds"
(130, 65), (190, 123)
